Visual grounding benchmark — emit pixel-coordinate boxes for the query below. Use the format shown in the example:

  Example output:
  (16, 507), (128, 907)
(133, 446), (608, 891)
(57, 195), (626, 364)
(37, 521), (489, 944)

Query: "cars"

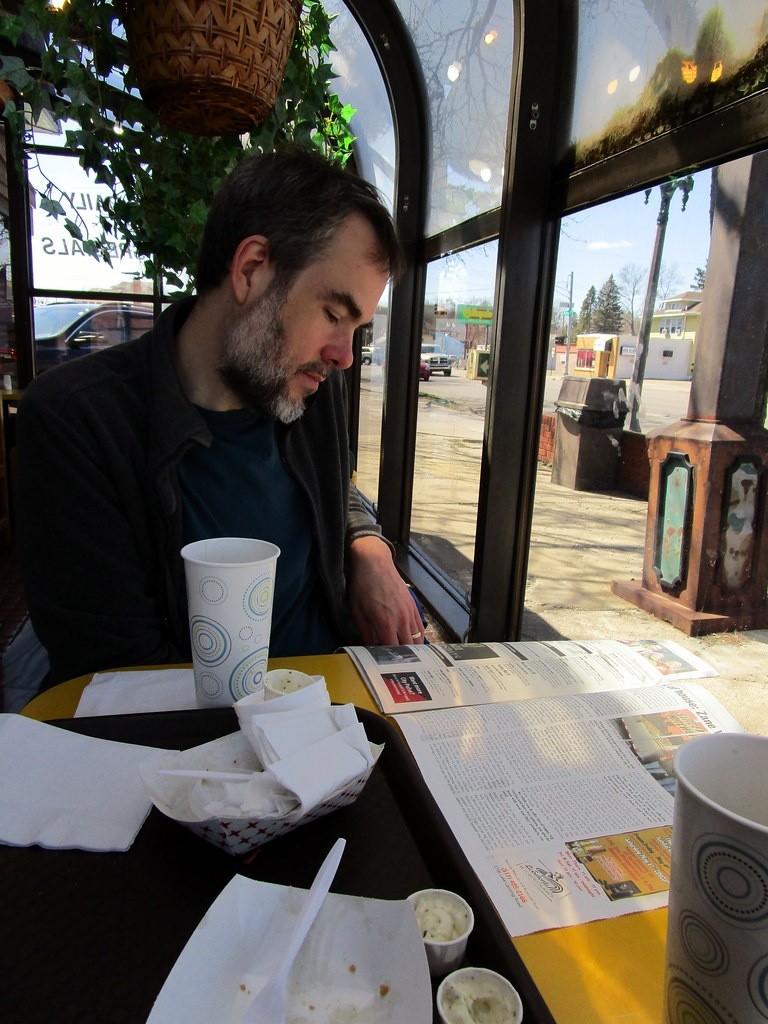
(381, 355), (430, 382)
(0, 301), (155, 381)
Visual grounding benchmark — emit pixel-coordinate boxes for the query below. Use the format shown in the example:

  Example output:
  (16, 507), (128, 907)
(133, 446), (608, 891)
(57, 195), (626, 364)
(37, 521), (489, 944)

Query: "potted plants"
(0, 0), (360, 303)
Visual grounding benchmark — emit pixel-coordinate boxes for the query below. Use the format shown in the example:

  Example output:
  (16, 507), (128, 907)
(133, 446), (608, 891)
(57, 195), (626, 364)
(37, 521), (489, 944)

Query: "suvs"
(362, 346), (382, 366)
(420, 343), (452, 378)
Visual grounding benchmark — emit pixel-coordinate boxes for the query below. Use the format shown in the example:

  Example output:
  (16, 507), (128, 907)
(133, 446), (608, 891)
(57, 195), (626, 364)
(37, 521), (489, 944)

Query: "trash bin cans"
(551, 375), (630, 492)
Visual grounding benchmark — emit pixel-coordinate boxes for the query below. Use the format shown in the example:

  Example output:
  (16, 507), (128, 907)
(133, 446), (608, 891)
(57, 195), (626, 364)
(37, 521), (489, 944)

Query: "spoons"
(237, 836), (347, 1023)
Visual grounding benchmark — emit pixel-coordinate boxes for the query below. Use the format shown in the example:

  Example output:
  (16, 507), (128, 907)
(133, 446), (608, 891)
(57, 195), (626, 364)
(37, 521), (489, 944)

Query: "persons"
(5, 151), (426, 677)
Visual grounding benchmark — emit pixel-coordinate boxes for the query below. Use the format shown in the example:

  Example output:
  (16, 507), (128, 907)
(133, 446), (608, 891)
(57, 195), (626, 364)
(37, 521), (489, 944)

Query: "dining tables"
(5, 656), (768, 1023)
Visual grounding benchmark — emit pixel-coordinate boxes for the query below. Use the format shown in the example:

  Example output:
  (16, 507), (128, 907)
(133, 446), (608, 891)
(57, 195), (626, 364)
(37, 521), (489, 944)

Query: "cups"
(178, 536), (282, 708)
(435, 968), (526, 1024)
(661, 733), (768, 1024)
(261, 666), (316, 702)
(405, 888), (475, 980)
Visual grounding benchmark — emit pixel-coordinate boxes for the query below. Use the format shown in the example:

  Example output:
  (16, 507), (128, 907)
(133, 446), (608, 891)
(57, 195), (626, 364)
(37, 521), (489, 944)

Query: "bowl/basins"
(138, 728), (386, 858)
(143, 874), (434, 1024)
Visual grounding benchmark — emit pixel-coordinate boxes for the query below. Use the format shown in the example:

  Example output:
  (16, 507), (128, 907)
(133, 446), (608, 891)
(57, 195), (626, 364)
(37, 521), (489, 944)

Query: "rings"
(412, 631), (422, 640)
